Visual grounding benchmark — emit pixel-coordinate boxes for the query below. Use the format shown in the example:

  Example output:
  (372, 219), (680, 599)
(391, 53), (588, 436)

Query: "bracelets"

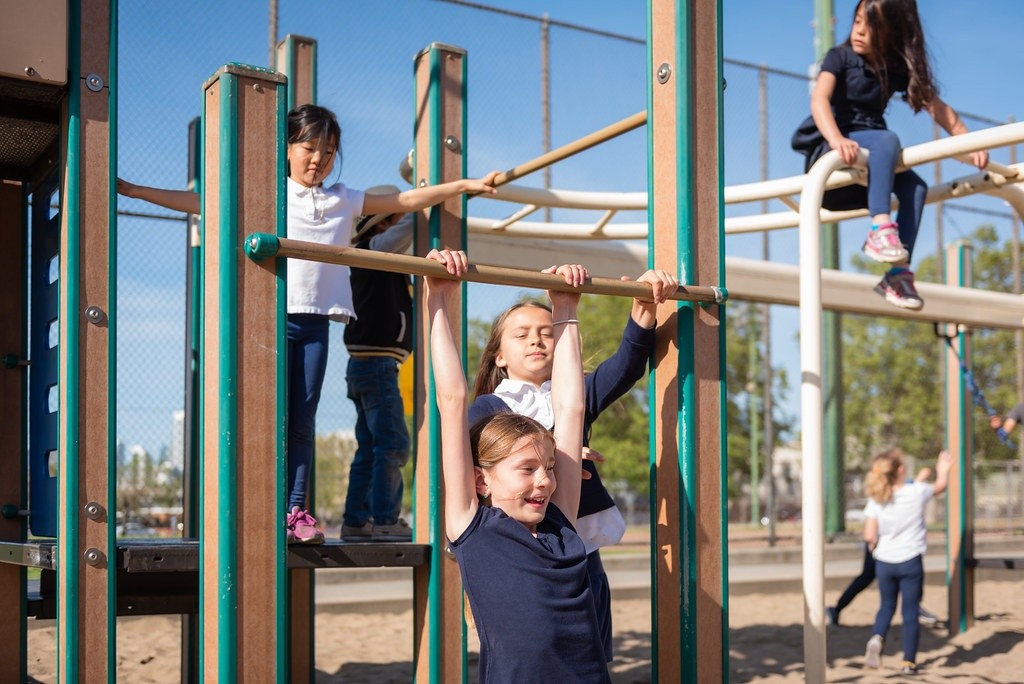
(552, 319), (579, 326)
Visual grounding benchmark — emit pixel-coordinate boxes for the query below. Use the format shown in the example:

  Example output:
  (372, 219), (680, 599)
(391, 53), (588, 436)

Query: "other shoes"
(866, 637), (882, 668)
(901, 662), (917, 675)
(337, 517), (372, 541)
(919, 606), (938, 624)
(284, 506), (326, 545)
(363, 515), (414, 542)
(825, 607), (839, 626)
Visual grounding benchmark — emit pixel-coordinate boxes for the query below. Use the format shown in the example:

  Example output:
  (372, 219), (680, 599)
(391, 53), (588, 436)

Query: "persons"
(339, 184), (415, 541)
(826, 450), (953, 676)
(423, 249), (613, 684)
(468, 269), (680, 663)
(990, 400), (1024, 440)
(117, 104), (501, 546)
(791, 0), (990, 309)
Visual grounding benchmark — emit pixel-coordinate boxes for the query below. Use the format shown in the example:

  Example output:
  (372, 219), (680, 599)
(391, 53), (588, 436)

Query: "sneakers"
(862, 222), (909, 266)
(874, 270), (924, 309)
